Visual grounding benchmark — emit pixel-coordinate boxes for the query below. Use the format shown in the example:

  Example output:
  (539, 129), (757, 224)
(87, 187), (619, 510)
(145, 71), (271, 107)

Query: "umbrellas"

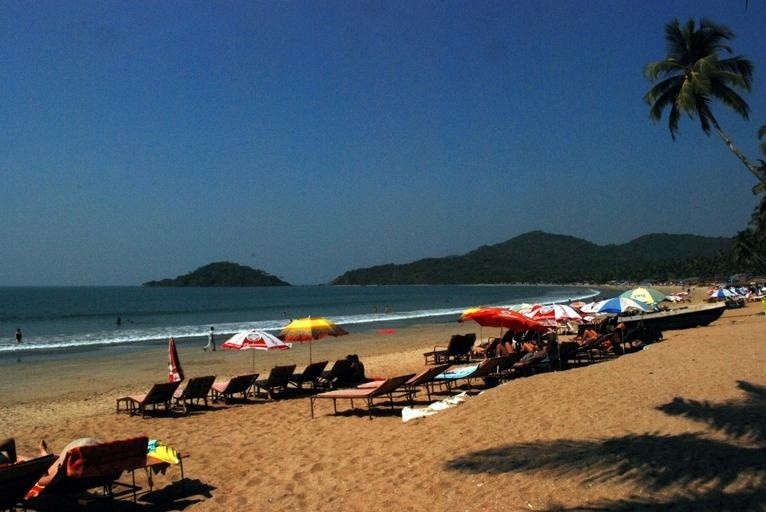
(277, 318), (349, 363)
(167, 336), (185, 384)
(219, 329), (292, 396)
(458, 306), (548, 336)
(619, 286), (669, 306)
(512, 301), (596, 321)
(592, 296), (654, 317)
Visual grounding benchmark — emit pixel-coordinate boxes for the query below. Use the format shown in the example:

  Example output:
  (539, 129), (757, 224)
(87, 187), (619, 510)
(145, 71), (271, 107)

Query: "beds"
(473, 338), (501, 353)
(430, 356), (507, 397)
(211, 373), (260, 405)
(320, 360), (352, 385)
(253, 364), (297, 403)
(310, 373), (417, 421)
(289, 360), (329, 395)
(568, 333), (615, 366)
(116, 379), (181, 419)
(12, 436), (148, 511)
(356, 362), (453, 408)
(466, 334), (476, 352)
(423, 336), (469, 365)
(172, 374), (217, 416)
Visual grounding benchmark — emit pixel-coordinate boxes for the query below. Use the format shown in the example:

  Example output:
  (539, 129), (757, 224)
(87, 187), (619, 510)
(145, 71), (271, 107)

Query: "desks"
(126, 449), (191, 504)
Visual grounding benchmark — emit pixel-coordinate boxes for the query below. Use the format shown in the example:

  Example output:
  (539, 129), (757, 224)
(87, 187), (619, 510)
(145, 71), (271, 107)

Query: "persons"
(202, 325), (217, 352)
(709, 286), (765, 307)
(344, 354), (365, 383)
(605, 323), (624, 353)
(18, 437), (106, 486)
(568, 296), (572, 303)
(115, 314), (133, 326)
(0, 437), (18, 464)
(522, 342), (537, 353)
(16, 328), (20, 343)
(496, 342), (515, 358)
(661, 304), (669, 310)
(593, 295), (604, 302)
(581, 329), (597, 342)
